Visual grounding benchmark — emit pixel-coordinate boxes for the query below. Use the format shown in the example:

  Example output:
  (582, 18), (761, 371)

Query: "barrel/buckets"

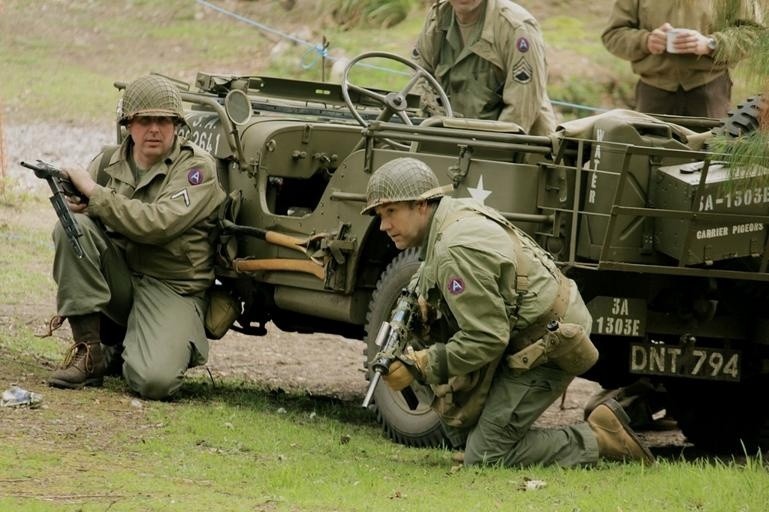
(577, 116), (695, 262)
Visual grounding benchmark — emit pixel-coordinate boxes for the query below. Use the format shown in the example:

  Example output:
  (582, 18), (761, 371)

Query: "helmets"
(359, 157), (445, 216)
(118, 74), (184, 126)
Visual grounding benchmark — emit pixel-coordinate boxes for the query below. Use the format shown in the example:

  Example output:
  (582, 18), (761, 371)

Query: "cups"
(665, 28), (689, 56)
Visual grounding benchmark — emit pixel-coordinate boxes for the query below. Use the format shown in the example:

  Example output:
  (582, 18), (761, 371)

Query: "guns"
(20, 160), (106, 257)
(361, 260), (441, 410)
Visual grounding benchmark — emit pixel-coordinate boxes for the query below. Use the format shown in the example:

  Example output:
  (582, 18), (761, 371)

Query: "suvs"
(112, 35), (767, 469)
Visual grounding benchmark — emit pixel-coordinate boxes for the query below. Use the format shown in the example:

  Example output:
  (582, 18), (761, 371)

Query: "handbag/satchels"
(426, 356), (501, 431)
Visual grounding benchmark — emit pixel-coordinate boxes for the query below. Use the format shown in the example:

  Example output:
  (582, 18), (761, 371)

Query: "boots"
(587, 398), (656, 468)
(48, 341), (106, 389)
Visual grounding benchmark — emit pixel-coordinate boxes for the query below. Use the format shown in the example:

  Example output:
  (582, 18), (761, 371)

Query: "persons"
(359, 157), (651, 468)
(600, 0), (768, 118)
(47, 73), (228, 401)
(409, 1), (554, 136)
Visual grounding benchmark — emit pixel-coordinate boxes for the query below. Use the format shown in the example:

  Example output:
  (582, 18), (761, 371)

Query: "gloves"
(382, 349), (429, 392)
